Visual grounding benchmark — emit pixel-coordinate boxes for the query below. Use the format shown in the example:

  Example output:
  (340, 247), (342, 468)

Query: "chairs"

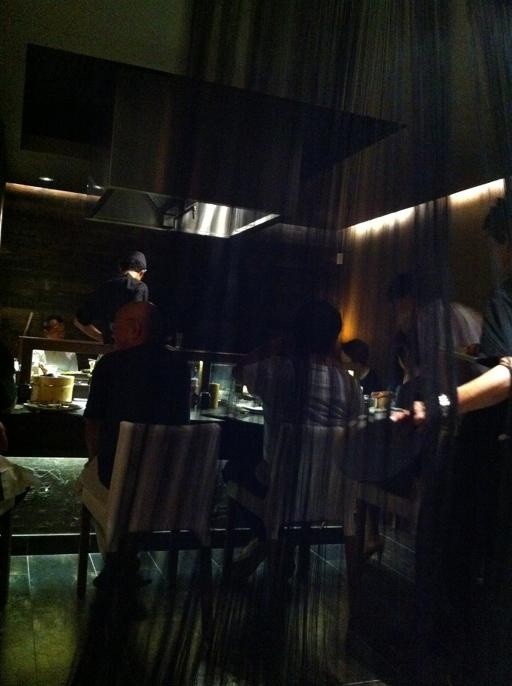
(77, 419), (223, 643)
(220, 422), (368, 640)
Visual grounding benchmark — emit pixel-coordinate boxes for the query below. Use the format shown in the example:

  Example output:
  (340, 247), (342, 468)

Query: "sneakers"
(93, 556), (151, 590)
(231, 539), (296, 594)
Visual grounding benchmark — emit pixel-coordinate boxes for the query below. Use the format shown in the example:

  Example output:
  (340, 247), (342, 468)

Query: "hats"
(122, 251), (146, 267)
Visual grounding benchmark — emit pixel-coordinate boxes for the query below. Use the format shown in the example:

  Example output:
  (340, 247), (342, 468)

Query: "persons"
(2, 198), (512, 590)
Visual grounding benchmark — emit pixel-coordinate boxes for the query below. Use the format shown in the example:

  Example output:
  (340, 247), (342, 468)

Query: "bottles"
(190, 378), (199, 409)
(198, 392), (210, 409)
(209, 383), (220, 409)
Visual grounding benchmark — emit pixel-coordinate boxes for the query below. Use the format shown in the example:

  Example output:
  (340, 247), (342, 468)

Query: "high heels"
(367, 539), (383, 560)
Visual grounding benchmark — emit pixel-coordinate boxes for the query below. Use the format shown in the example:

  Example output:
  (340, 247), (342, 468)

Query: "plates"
(242, 404), (264, 415)
(23, 400), (82, 413)
